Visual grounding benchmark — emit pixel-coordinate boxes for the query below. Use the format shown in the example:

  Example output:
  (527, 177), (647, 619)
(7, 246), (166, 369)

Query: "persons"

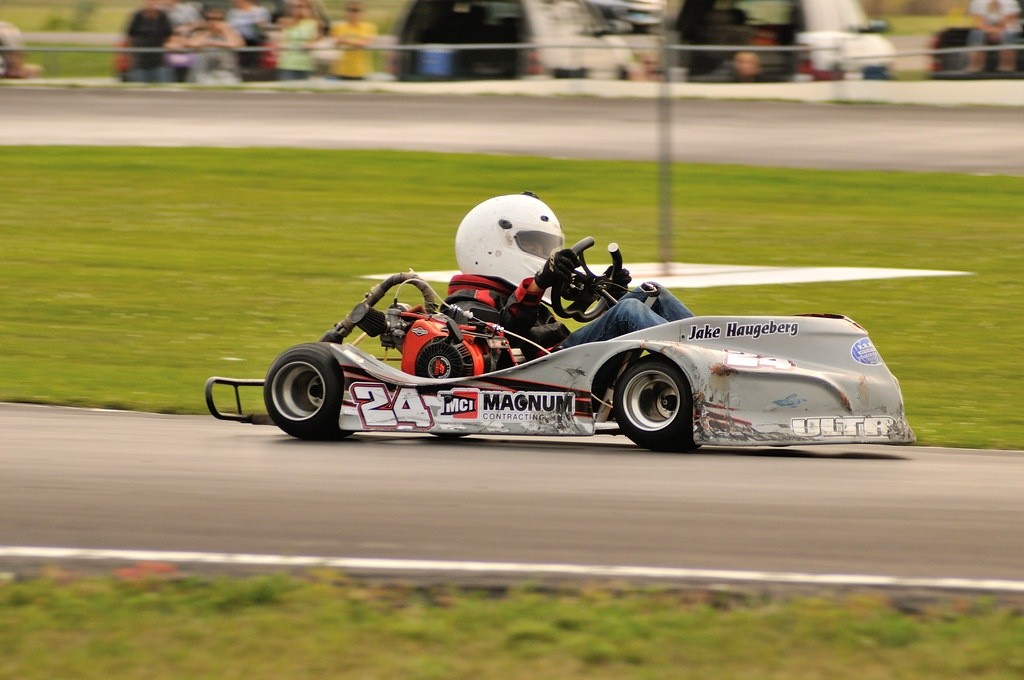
(440, 193), (696, 365)
(0, 0), (1024, 88)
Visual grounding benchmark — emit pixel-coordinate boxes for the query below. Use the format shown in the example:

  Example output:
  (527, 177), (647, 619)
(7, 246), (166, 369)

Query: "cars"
(114, 0), (332, 86)
(393, 0), (662, 80)
(658, 1), (897, 83)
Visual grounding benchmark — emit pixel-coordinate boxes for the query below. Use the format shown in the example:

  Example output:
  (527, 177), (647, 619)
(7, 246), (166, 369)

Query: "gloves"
(602, 265), (632, 291)
(534, 248), (582, 291)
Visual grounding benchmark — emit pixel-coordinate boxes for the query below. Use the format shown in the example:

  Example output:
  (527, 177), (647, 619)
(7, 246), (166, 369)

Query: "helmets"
(454, 190), (565, 307)
